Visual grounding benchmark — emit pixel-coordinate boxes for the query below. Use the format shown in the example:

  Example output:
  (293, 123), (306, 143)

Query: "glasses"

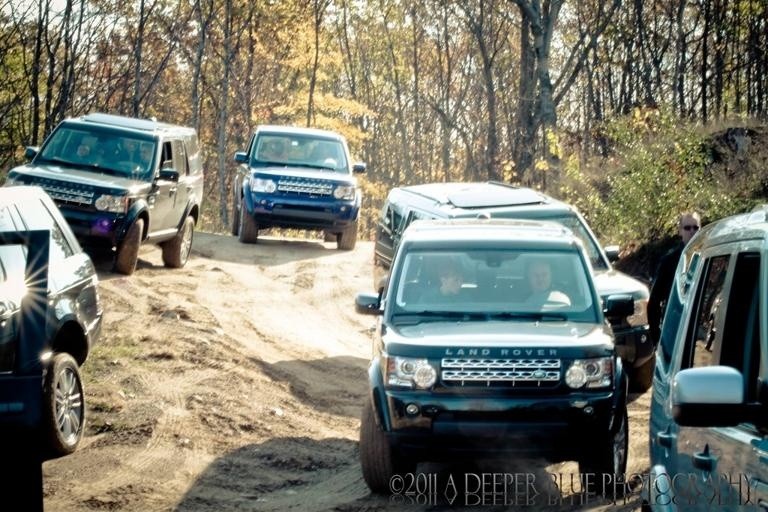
(683, 225), (699, 231)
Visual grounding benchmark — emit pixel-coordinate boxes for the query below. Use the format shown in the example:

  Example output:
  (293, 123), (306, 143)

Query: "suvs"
(0, 181), (105, 464)
(353, 212), (638, 500)
(7, 112), (204, 276)
(367, 173), (656, 393)
(228, 123), (370, 253)
(644, 201), (768, 511)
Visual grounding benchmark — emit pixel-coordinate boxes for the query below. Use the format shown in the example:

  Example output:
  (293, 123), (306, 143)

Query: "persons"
(310, 141), (340, 165)
(258, 141), (289, 160)
(412, 261), (475, 306)
(506, 259), (580, 306)
(73, 140), (152, 170)
(646, 212), (703, 344)
